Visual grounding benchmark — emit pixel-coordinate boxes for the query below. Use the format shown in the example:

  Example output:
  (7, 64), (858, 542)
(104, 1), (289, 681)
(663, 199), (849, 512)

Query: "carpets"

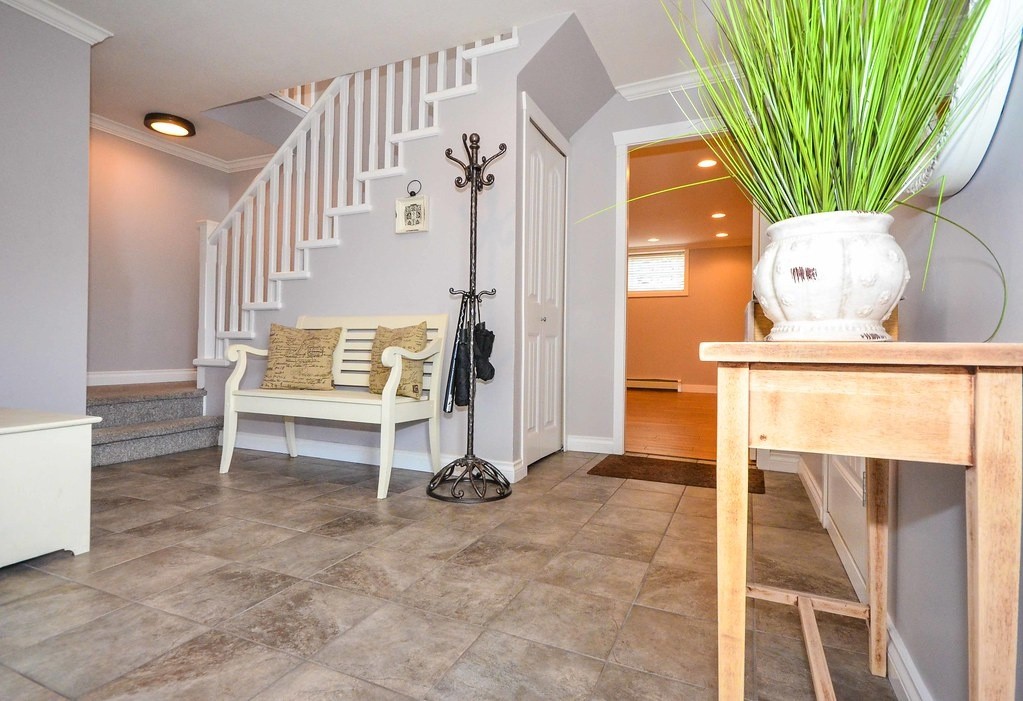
(586, 454), (765, 494)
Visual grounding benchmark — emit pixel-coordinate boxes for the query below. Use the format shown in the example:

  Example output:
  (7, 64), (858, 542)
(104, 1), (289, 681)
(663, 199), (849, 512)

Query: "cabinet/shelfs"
(0, 408), (103, 568)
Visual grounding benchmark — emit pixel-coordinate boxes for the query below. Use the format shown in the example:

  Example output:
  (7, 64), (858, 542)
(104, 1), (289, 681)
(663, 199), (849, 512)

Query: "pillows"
(369, 321), (427, 400)
(260, 324), (342, 392)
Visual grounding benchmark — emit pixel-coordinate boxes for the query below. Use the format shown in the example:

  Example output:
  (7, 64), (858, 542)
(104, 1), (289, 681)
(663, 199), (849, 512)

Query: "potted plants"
(575, 0), (1007, 342)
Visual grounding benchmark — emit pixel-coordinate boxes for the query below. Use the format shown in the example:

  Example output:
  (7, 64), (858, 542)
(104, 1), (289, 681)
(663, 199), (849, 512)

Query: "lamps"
(144, 112), (196, 138)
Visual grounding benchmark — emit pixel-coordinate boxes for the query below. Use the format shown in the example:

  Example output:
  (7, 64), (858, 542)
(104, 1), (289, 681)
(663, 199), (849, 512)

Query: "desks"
(700, 342), (1022, 701)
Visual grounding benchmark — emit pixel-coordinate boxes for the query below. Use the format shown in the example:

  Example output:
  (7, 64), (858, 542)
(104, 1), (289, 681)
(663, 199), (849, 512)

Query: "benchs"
(219, 313), (449, 498)
